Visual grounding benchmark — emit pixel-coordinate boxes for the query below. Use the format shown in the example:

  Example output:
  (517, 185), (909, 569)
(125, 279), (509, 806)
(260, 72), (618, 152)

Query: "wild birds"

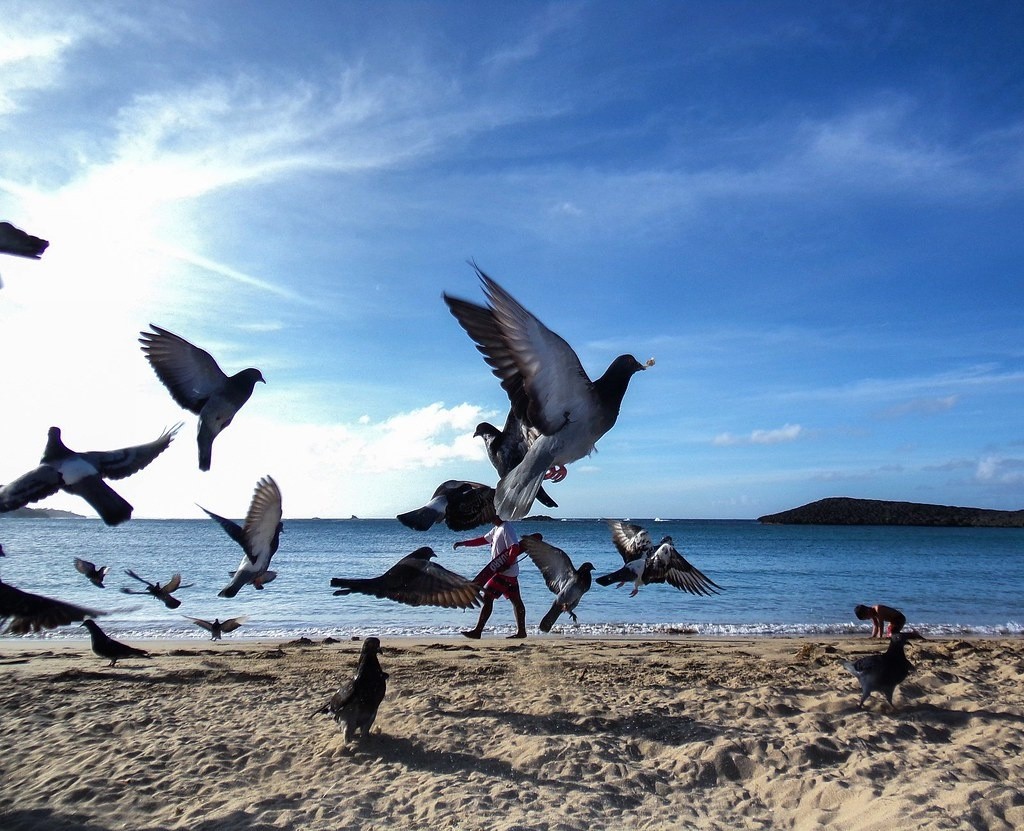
(194, 473), (287, 599)
(329, 546), (492, 610)
(1, 419), (184, 525)
(79, 619), (153, 669)
(329, 636), (388, 745)
(181, 614), (250, 642)
(593, 519), (726, 599)
(73, 555), (111, 589)
(120, 569), (196, 610)
(139, 323), (266, 472)
(0, 222), (50, 261)
(836, 633), (914, 712)
(519, 534), (596, 632)
(4, 583), (109, 636)
(394, 255), (647, 531)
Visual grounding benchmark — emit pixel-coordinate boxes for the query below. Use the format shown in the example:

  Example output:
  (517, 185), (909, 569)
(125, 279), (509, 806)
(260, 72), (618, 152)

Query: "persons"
(453, 518), (527, 639)
(854, 605), (926, 640)
